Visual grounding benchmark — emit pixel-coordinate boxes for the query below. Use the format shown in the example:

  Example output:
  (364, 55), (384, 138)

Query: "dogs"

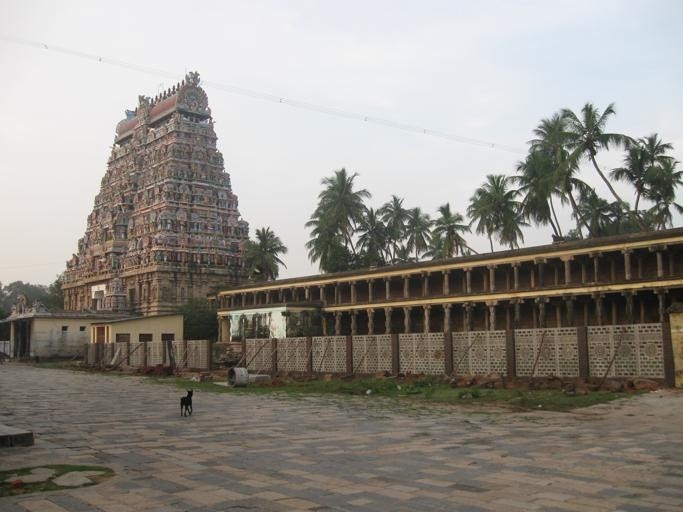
(180, 390), (193, 417)
